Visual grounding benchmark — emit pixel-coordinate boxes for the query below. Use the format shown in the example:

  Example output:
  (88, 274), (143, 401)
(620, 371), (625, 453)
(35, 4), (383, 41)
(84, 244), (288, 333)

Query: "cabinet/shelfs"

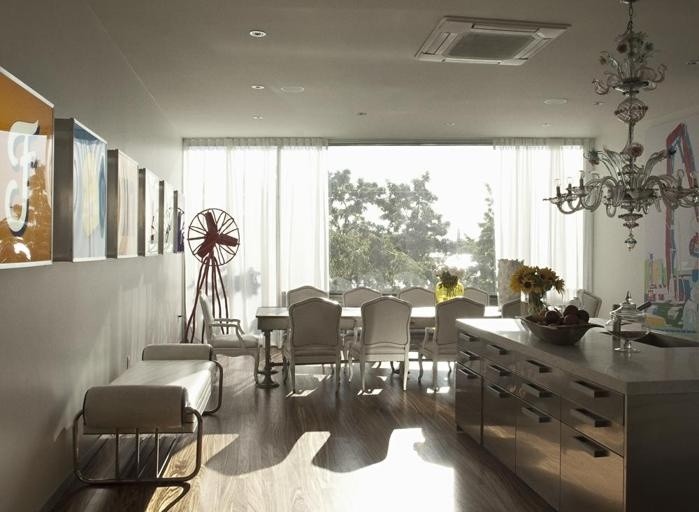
(455, 328), (626, 512)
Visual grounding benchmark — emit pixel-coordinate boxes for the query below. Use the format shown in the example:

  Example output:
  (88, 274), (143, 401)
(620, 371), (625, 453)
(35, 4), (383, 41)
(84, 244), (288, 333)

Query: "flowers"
(434, 266), (466, 295)
(509, 266), (565, 309)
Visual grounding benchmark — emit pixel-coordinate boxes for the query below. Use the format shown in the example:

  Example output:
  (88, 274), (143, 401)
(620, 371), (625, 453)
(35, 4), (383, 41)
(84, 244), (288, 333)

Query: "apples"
(545, 305), (589, 326)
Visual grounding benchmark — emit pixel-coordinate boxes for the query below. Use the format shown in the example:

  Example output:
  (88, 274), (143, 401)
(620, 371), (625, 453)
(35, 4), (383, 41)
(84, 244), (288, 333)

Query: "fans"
(184, 208), (240, 343)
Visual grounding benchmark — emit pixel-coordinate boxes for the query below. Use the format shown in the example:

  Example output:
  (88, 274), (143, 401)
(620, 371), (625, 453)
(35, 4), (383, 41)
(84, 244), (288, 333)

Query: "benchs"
(73, 344), (223, 485)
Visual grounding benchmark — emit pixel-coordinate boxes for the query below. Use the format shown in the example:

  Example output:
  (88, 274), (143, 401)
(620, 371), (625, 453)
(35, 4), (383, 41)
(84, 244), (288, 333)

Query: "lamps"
(542, 0), (699, 249)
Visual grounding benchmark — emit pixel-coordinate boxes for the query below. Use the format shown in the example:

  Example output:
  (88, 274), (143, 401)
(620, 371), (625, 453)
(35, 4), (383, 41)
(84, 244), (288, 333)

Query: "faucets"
(612, 302), (651, 348)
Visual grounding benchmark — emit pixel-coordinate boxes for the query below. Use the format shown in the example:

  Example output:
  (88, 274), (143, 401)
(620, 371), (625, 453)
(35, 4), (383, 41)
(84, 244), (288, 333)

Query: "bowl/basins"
(520, 316), (604, 346)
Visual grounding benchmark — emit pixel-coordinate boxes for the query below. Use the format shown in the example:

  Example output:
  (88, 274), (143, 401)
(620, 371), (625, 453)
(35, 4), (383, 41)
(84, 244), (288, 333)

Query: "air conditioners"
(413, 14), (574, 68)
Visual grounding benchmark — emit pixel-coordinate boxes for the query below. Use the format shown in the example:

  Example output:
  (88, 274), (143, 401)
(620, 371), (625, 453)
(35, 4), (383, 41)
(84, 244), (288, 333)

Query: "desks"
(256, 306), (502, 389)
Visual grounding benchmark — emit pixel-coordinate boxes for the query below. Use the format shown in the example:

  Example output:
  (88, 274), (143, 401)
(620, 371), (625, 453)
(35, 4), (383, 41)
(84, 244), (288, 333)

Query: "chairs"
(200, 294), (260, 384)
(282, 286), (601, 393)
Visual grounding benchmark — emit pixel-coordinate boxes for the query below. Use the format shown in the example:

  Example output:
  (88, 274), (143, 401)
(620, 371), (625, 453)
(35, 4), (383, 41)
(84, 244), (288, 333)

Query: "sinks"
(600, 330), (699, 348)
